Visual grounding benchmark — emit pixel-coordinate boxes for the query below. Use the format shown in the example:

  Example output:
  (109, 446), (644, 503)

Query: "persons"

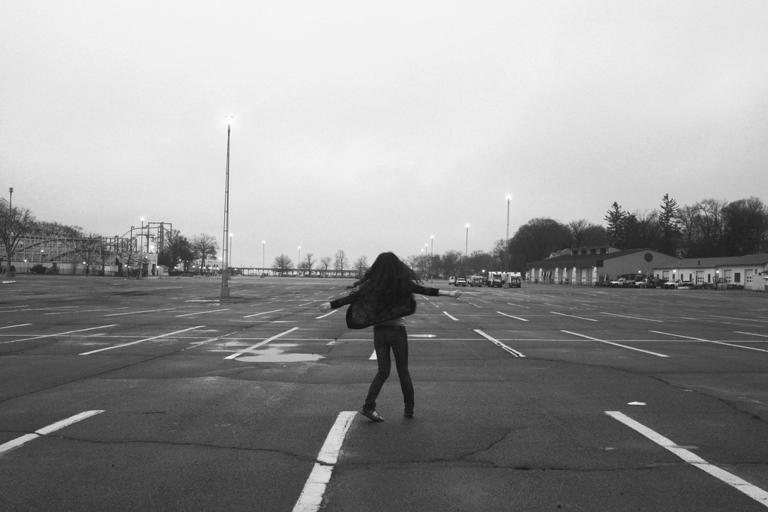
(319, 250), (463, 423)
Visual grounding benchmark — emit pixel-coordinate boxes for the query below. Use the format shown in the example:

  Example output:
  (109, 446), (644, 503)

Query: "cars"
(609, 274), (679, 288)
(448, 271), (521, 288)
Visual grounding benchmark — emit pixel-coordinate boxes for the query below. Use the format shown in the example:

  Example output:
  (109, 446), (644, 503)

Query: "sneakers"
(360, 408), (413, 423)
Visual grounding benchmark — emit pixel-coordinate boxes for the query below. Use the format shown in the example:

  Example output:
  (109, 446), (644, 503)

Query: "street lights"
(261, 240), (265, 267)
(298, 247), (301, 265)
(229, 232), (233, 265)
(506, 193), (512, 276)
(466, 224), (469, 256)
(136, 218), (145, 280)
(421, 236), (434, 256)
